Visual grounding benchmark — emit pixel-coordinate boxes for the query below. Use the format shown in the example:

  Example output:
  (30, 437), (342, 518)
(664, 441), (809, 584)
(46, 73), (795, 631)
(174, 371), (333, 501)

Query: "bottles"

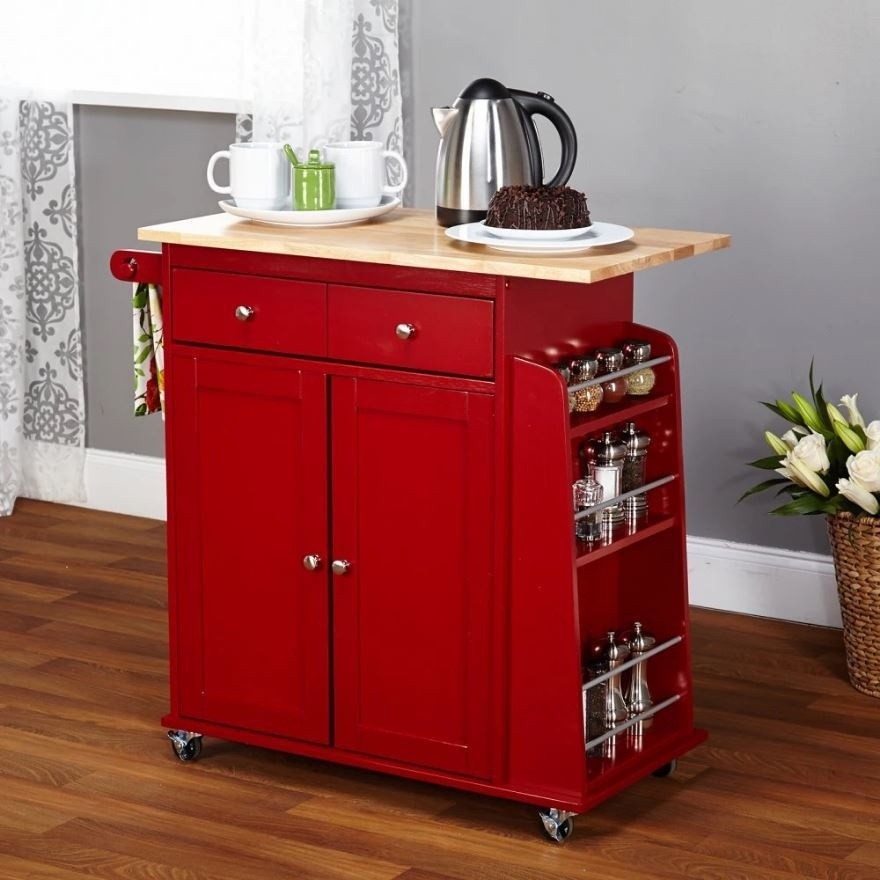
(623, 621), (657, 711)
(555, 365), (576, 414)
(572, 474), (603, 542)
(596, 631), (628, 722)
(594, 349), (628, 403)
(621, 341), (655, 395)
(568, 355), (603, 412)
(615, 422), (650, 519)
(583, 432), (624, 523)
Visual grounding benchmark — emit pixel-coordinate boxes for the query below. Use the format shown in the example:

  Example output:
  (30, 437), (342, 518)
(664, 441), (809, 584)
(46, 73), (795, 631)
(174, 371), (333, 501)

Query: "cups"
(293, 150), (335, 210)
(322, 141), (408, 210)
(207, 142), (291, 210)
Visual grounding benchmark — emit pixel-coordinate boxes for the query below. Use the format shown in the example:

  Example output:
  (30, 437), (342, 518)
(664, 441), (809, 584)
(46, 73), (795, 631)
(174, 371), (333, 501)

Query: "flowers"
(734, 352), (880, 517)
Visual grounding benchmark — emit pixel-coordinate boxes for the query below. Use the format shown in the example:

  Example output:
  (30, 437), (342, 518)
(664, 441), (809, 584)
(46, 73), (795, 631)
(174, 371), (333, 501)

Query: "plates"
(218, 195), (402, 227)
(444, 219), (633, 253)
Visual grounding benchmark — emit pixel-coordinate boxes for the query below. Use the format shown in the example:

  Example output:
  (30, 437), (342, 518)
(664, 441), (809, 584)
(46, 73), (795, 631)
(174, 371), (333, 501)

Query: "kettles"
(430, 78), (577, 228)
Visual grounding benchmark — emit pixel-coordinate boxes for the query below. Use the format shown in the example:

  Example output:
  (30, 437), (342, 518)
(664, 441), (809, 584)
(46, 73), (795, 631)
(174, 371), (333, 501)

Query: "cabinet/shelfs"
(111, 206), (731, 845)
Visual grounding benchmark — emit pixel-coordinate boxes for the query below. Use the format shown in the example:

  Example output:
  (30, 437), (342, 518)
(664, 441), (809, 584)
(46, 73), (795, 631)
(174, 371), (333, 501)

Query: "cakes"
(484, 185), (591, 230)
